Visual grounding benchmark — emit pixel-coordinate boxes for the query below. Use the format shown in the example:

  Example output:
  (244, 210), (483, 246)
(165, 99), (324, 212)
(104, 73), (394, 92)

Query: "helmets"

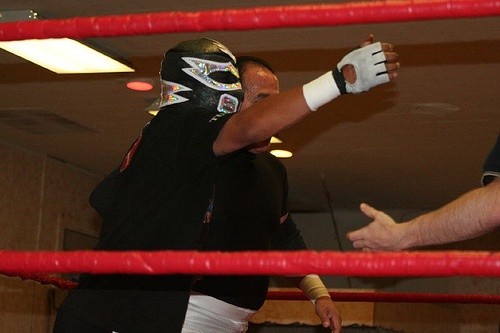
(145, 36), (245, 117)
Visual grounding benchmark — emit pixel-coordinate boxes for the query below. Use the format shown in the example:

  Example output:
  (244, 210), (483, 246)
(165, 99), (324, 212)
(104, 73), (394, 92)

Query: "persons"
(346, 139), (500, 252)
(53, 35), (401, 333)
